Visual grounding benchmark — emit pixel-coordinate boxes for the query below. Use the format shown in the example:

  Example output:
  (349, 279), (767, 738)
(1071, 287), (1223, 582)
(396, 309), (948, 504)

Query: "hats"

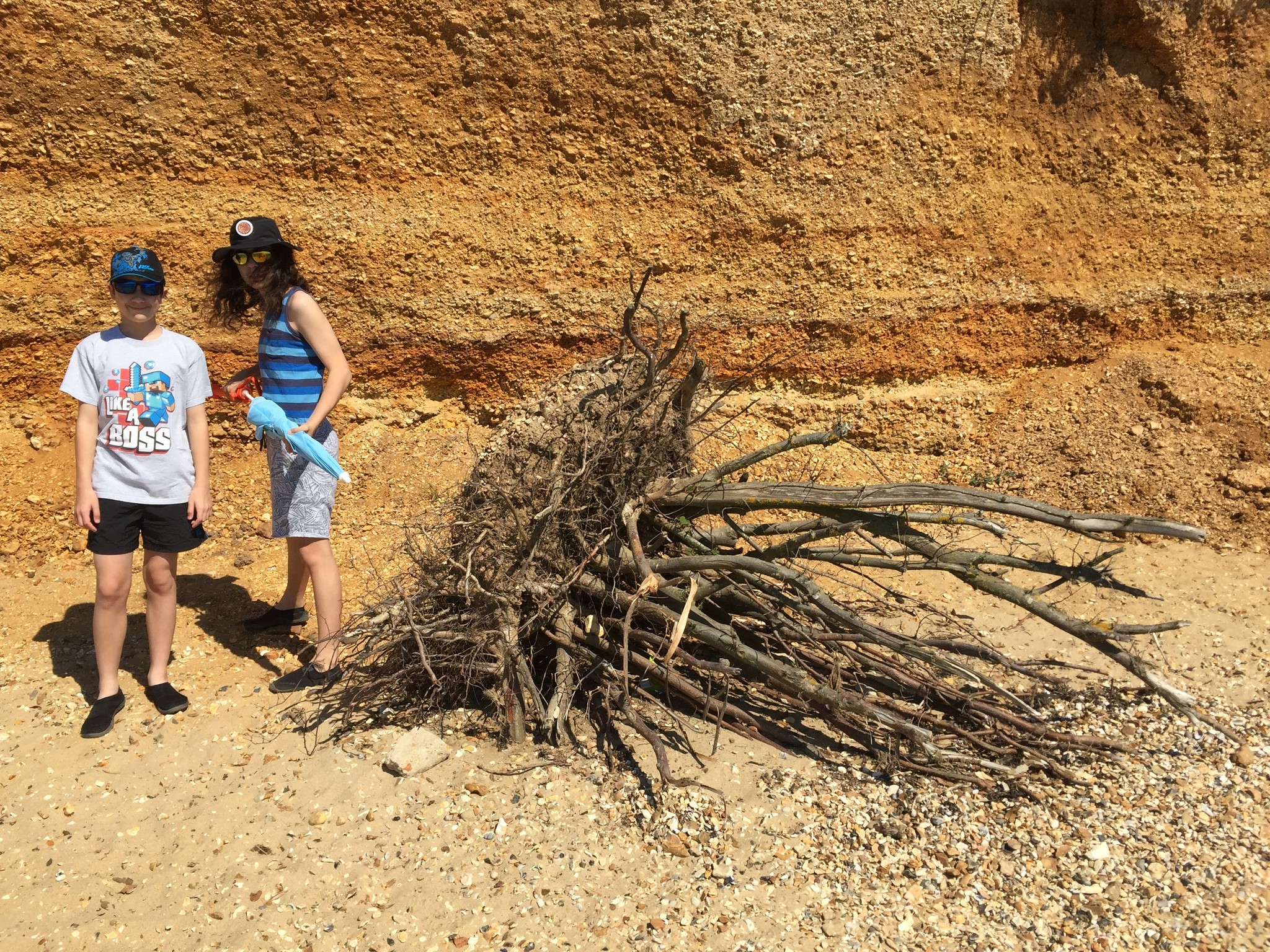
(107, 245), (166, 283)
(212, 216), (304, 264)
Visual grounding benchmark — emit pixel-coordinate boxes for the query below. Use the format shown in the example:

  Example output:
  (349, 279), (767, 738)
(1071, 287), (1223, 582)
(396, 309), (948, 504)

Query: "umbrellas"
(208, 383), (352, 484)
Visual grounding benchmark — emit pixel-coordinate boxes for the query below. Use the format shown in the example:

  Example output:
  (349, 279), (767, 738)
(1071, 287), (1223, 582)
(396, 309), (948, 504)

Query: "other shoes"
(80, 686), (125, 738)
(245, 606), (307, 630)
(146, 682), (189, 714)
(269, 662), (342, 692)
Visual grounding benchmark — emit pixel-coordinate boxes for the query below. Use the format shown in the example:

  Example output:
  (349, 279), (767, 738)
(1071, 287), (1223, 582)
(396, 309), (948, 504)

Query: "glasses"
(113, 279), (164, 296)
(233, 250), (274, 265)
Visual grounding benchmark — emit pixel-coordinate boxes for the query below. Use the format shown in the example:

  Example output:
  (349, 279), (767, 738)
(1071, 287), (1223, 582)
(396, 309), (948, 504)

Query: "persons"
(59, 245), (213, 738)
(200, 216), (352, 693)
